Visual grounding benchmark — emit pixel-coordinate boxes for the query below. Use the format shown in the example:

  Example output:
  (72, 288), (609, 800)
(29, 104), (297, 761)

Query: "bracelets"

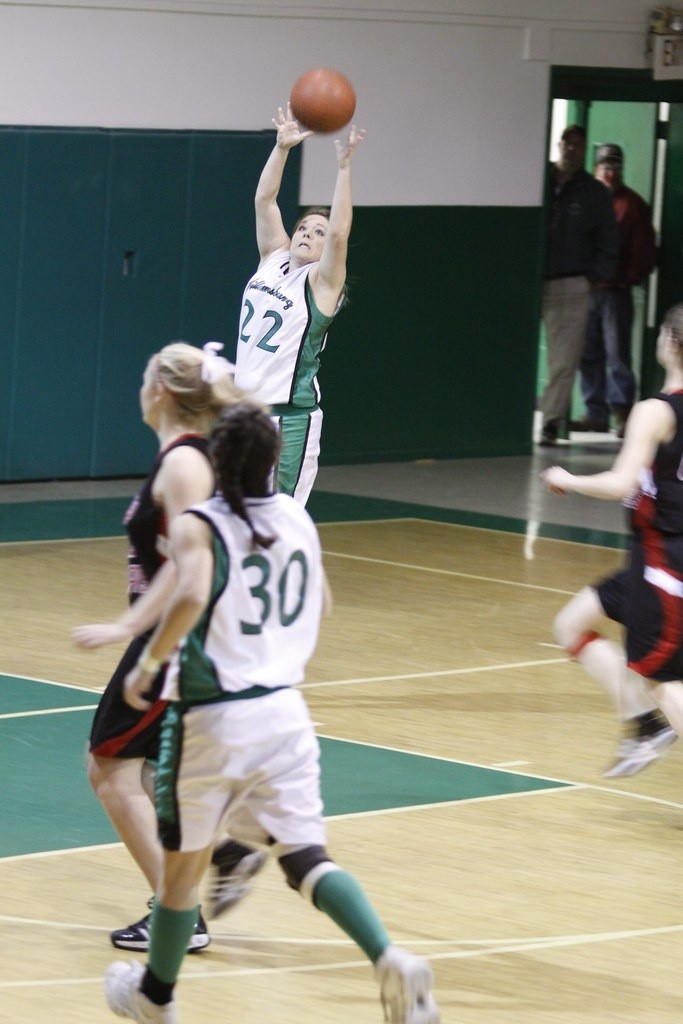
(137, 646), (161, 672)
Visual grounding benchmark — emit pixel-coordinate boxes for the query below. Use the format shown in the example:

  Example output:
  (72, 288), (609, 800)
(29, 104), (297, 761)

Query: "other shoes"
(539, 424), (557, 446)
(568, 416), (609, 432)
(614, 404), (632, 437)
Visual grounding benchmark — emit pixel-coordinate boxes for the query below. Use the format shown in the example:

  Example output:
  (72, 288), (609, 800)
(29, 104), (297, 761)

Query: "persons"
(234, 101), (367, 507)
(537, 124), (683, 782)
(68, 341), (271, 952)
(104, 403), (443, 1024)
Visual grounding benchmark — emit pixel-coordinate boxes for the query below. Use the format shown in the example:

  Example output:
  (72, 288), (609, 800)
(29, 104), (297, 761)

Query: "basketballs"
(290, 67), (358, 134)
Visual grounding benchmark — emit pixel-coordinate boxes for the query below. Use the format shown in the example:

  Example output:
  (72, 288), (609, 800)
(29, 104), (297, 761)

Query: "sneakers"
(104, 959), (180, 1024)
(207, 843), (268, 919)
(372, 946), (442, 1024)
(110, 896), (210, 951)
(604, 708), (678, 777)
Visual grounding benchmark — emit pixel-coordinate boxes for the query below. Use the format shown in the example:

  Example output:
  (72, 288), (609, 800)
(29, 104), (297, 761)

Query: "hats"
(561, 124), (586, 139)
(596, 144), (623, 163)
